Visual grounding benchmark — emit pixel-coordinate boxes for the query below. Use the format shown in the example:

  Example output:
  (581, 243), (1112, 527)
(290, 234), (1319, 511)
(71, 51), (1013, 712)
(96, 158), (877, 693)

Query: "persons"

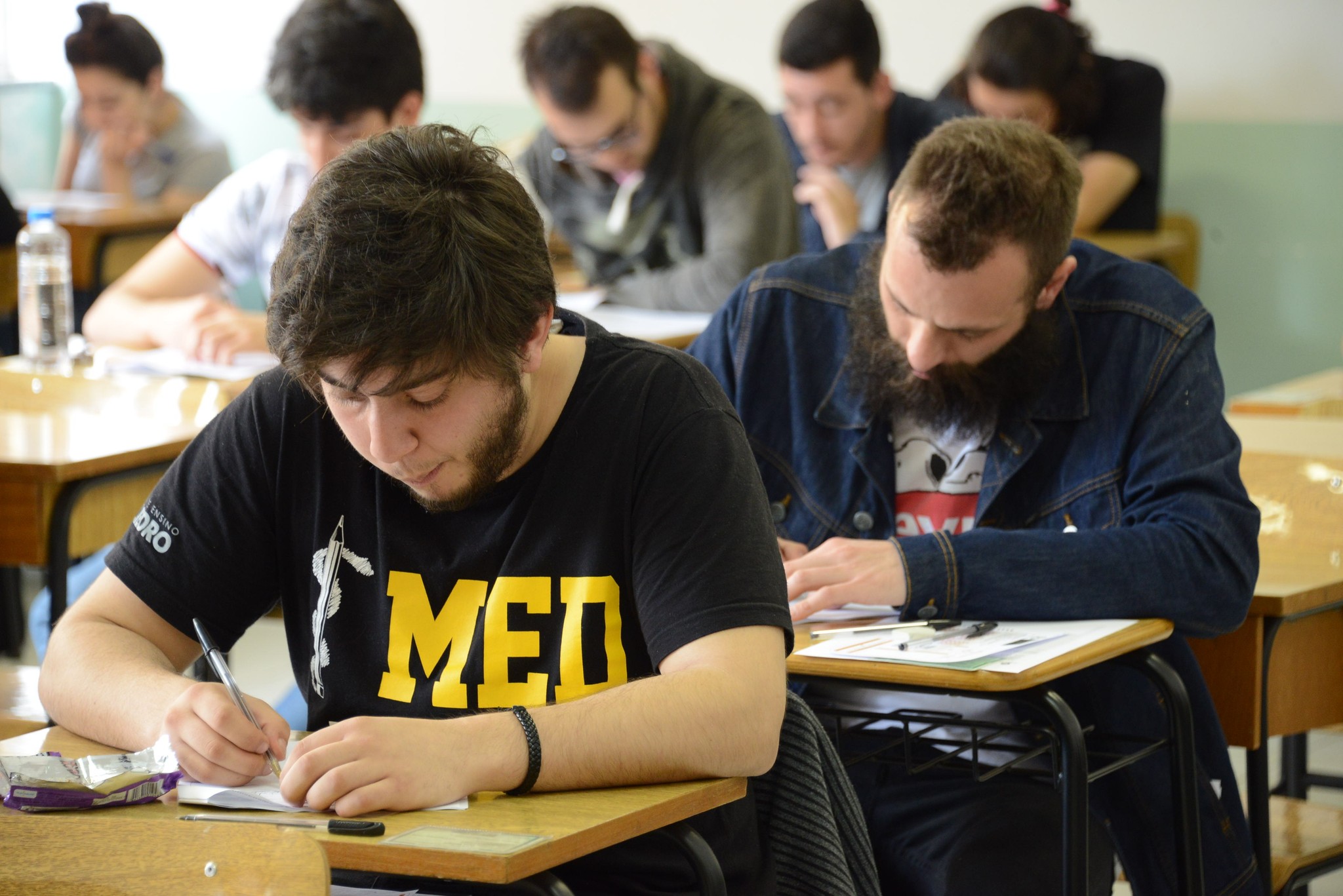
(511, 5), (801, 312)
(85, 0), (425, 367)
(683, 117), (1260, 896)
(936, 0), (1155, 231)
(58, 0), (233, 205)
(772, 0), (934, 255)
(38, 125), (795, 896)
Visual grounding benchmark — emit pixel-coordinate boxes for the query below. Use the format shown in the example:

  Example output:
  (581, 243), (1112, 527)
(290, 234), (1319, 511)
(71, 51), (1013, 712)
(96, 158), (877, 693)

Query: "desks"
(0, 188), (1343, 896)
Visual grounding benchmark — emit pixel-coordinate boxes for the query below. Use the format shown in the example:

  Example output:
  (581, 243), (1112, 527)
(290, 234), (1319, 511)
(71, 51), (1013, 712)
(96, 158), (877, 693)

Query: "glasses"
(552, 94), (642, 164)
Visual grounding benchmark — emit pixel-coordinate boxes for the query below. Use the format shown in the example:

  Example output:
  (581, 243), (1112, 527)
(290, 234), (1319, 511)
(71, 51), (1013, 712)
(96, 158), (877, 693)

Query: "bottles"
(15, 210), (75, 366)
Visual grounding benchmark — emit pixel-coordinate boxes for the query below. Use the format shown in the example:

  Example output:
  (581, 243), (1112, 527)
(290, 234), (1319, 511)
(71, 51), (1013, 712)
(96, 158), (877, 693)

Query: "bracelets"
(504, 705), (541, 795)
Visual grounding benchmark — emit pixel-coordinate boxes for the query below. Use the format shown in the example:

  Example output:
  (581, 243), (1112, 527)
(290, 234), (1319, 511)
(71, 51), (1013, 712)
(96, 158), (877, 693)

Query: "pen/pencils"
(810, 620), (962, 640)
(193, 617), (282, 778)
(176, 814), (386, 837)
(898, 622), (998, 651)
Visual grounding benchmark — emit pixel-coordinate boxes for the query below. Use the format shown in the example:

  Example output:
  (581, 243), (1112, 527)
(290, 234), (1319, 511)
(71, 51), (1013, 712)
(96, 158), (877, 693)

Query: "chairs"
(555, 686), (883, 896)
(0, 814), (331, 896)
